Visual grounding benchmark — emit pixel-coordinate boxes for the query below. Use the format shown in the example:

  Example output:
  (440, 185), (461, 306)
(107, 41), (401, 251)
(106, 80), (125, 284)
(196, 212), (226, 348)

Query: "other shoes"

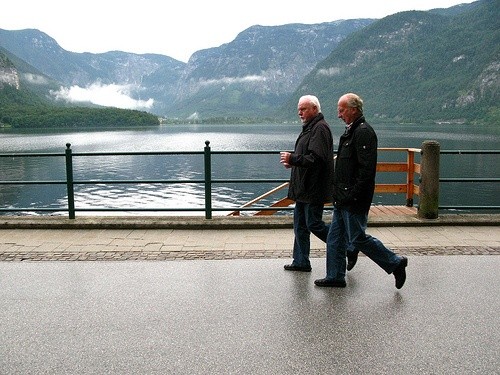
(314, 277), (347, 288)
(284, 260), (312, 272)
(393, 256), (407, 289)
(347, 248), (360, 271)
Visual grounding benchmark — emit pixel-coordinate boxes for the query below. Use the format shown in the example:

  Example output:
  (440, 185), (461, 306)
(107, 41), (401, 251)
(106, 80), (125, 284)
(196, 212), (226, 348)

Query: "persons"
(314, 92), (408, 290)
(279, 94), (360, 272)
